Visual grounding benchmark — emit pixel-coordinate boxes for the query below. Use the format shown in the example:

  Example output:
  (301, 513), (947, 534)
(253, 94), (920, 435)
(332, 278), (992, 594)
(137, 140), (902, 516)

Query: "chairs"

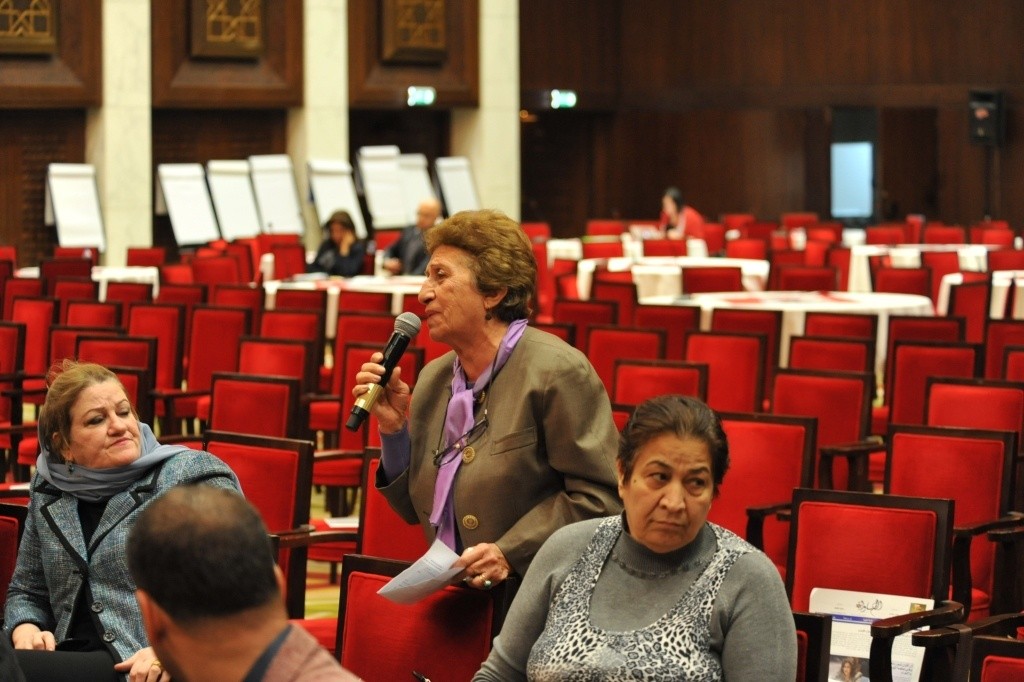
(0, 211), (1022, 681)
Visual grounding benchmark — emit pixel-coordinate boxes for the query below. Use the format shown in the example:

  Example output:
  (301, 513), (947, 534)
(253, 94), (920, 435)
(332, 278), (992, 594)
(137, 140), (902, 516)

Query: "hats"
(324, 211), (355, 232)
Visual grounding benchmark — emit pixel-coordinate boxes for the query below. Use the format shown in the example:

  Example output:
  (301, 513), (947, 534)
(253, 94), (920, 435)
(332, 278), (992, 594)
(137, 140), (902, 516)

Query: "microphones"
(345, 311), (421, 431)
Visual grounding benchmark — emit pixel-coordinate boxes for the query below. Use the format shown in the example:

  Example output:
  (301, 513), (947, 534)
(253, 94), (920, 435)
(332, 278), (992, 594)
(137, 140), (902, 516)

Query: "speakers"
(968, 86), (1007, 146)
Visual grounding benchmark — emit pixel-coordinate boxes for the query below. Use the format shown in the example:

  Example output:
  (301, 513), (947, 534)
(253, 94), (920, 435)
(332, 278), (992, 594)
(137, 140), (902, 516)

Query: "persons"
(835, 659), (857, 682)
(307, 210), (364, 278)
(351, 207), (629, 642)
(3, 356), (252, 682)
(127, 484), (366, 682)
(377, 200), (444, 279)
(657, 188), (707, 239)
(472, 393), (798, 681)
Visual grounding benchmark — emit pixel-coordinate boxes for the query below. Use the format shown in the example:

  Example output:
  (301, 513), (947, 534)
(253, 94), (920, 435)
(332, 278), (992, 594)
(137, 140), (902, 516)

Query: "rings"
(479, 574), (494, 589)
(150, 660), (165, 672)
